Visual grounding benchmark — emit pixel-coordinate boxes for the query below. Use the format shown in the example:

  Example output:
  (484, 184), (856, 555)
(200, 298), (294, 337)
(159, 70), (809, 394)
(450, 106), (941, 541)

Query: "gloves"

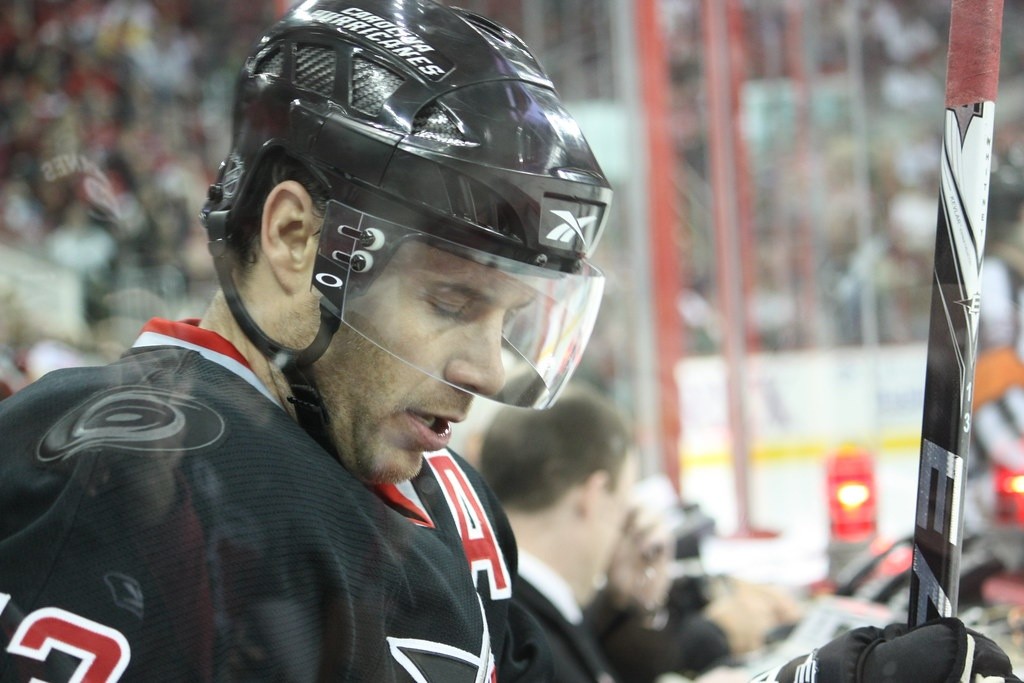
(750, 618), (1021, 683)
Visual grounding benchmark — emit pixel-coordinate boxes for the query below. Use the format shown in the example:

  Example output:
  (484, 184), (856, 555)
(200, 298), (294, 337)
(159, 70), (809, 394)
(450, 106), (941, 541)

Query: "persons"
(0, 0), (614, 683)
(470, 379), (805, 683)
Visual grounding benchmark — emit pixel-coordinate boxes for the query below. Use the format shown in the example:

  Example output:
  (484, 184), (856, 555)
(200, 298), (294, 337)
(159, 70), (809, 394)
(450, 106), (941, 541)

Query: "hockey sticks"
(905, 0), (1012, 631)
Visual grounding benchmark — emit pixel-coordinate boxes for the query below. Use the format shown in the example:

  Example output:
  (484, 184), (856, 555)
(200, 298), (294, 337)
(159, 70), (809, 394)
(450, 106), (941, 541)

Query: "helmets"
(198, 1), (616, 371)
